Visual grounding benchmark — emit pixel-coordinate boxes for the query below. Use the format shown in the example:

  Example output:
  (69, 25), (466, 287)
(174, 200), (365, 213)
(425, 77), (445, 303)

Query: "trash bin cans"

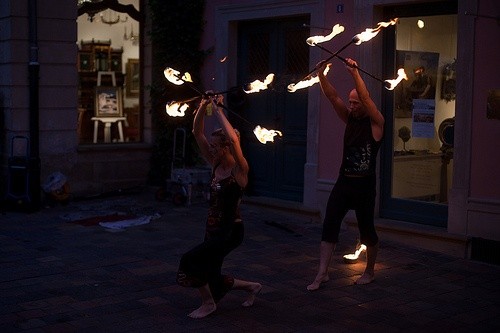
(0, 136), (41, 214)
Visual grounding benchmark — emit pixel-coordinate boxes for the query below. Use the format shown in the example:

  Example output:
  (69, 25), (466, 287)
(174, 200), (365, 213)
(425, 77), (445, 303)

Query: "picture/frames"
(126, 59), (140, 97)
(95, 87), (123, 116)
(77, 51), (95, 72)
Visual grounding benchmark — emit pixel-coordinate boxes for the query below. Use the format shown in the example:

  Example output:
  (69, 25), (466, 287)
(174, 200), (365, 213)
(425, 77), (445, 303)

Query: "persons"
(167, 90), (262, 318)
(307, 58), (384, 290)
